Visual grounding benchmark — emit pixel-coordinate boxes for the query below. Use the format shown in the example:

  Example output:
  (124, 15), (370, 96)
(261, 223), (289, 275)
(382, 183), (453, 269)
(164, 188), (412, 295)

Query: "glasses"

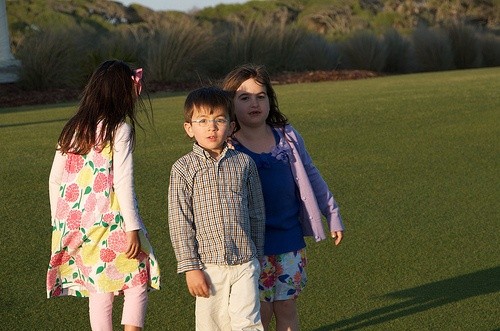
(191, 117), (232, 126)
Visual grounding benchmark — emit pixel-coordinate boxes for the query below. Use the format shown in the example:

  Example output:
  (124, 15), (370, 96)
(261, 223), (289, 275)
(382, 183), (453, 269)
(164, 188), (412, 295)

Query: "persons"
(168, 87), (267, 331)
(219, 64), (344, 330)
(45, 59), (161, 330)
